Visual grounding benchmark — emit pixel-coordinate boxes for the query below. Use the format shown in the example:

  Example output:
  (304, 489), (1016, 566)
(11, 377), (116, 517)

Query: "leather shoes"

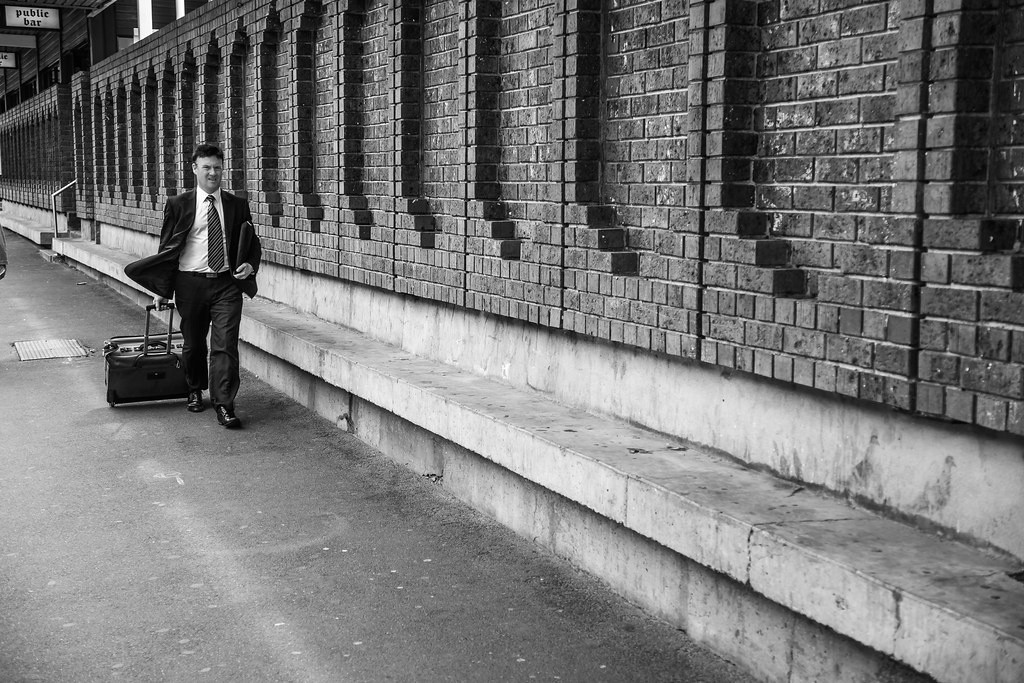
(186, 387), (204, 412)
(213, 405), (239, 427)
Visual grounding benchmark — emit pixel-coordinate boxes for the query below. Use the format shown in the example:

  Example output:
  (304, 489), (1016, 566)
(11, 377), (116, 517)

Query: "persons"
(125, 145), (262, 428)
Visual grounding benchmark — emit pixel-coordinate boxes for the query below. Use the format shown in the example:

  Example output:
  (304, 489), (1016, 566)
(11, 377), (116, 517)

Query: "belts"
(188, 271), (230, 279)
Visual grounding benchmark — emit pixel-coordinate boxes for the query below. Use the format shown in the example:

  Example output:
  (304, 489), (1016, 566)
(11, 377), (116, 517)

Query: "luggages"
(103, 304), (189, 407)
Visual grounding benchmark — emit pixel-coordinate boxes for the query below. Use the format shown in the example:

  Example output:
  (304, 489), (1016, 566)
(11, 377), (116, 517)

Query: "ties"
(206, 195), (224, 272)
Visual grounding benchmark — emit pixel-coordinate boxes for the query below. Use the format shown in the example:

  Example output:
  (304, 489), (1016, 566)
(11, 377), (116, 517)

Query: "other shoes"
(0, 263), (6, 278)
